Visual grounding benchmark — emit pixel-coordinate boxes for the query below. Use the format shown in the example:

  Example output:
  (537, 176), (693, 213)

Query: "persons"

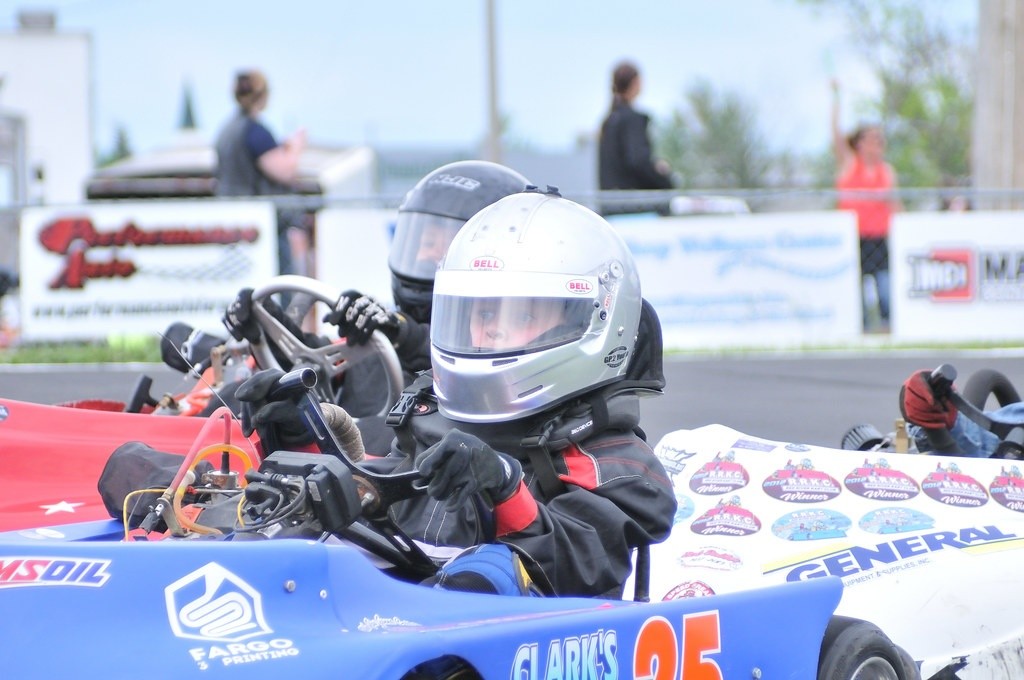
(900, 370), (1024, 457)
(599, 65), (674, 216)
(212, 68), (304, 279)
(188, 162), (678, 604)
(837, 127), (906, 330)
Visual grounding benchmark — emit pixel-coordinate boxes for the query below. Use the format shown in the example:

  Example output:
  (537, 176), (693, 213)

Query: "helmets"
(429, 193), (643, 423)
(388, 161), (542, 308)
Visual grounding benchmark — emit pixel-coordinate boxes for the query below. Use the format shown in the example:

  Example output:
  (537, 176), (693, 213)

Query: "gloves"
(223, 289), (304, 346)
(416, 427), (520, 513)
(323, 291), (399, 347)
(234, 369), (315, 446)
(904, 369), (958, 431)
